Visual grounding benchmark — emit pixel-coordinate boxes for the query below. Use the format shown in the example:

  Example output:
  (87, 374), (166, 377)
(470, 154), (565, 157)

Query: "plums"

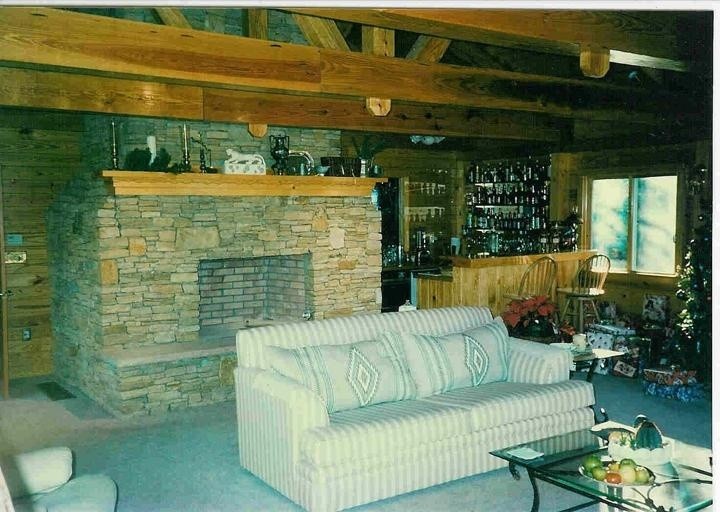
(606, 473), (622, 484)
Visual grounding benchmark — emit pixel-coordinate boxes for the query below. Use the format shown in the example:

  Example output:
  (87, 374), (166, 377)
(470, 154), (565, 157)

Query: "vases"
(525, 322), (555, 335)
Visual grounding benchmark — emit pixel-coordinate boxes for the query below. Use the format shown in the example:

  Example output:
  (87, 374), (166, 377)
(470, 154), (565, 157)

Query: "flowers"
(500, 294), (555, 328)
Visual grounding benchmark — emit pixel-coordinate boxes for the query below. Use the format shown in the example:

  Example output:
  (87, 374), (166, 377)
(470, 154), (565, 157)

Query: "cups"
(315, 165), (331, 176)
(296, 163), (306, 176)
(573, 333), (587, 353)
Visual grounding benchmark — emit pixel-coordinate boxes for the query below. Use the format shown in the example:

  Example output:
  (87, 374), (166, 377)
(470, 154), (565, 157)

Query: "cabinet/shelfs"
(403, 175), (449, 252)
(463, 179), (548, 233)
(382, 266), (411, 315)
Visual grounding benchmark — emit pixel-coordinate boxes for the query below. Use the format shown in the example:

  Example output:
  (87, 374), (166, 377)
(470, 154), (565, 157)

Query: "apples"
(618, 465), (650, 483)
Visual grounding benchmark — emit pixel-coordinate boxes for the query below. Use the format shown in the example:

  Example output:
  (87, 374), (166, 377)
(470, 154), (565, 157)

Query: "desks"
(570, 347), (626, 423)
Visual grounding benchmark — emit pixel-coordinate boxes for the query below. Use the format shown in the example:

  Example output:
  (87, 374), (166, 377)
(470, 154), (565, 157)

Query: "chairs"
(559, 253), (612, 332)
(0, 446), (120, 512)
(505, 254), (559, 317)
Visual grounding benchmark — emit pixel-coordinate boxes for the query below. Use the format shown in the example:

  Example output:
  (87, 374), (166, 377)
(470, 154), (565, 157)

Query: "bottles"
(410, 208), (445, 223)
(465, 157), (549, 231)
(408, 182), (444, 196)
(465, 231), (534, 257)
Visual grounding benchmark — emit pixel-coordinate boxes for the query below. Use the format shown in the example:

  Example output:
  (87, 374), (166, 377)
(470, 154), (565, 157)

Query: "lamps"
(410, 134), (447, 146)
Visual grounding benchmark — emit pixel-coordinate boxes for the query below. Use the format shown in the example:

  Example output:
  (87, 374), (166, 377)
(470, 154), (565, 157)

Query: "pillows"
(256, 336), (414, 413)
(386, 315), (513, 396)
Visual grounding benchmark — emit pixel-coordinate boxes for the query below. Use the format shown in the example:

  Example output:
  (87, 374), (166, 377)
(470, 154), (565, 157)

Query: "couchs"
(231, 304), (597, 512)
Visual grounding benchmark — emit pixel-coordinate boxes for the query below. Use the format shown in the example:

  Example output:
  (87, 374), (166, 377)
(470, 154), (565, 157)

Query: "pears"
(608, 462), (619, 470)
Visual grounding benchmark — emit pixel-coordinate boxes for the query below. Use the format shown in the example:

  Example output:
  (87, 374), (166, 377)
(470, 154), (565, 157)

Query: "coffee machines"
(414, 227), (432, 267)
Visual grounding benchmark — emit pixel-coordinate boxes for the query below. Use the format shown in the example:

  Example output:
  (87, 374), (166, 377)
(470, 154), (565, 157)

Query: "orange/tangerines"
(585, 454), (607, 481)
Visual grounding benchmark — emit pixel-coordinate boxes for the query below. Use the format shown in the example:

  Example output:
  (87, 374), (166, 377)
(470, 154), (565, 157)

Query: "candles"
(146, 135), (156, 167)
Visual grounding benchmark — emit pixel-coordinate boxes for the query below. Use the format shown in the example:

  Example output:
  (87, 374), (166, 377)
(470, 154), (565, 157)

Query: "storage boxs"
(610, 335), (652, 379)
(587, 322), (636, 375)
(319, 156), (362, 178)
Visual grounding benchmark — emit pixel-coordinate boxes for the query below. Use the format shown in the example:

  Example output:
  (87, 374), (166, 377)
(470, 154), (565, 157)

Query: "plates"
(577, 461), (654, 488)
